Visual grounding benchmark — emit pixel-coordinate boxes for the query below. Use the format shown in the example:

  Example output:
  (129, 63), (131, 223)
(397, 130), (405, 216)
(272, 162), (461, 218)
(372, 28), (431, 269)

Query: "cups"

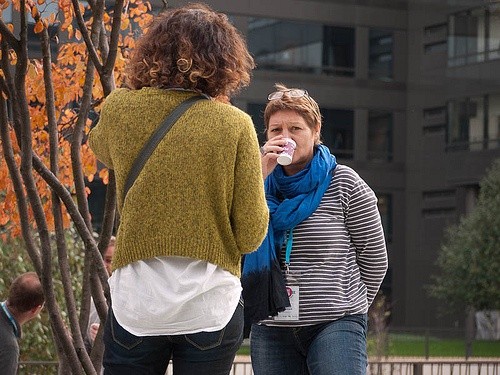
(277, 138), (297, 165)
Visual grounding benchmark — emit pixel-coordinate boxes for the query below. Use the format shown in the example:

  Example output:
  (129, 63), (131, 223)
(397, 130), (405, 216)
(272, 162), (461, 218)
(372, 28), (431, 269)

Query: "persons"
(82, 235), (118, 347)
(88, 3), (269, 375)
(243, 82), (389, 375)
(0, 272), (46, 375)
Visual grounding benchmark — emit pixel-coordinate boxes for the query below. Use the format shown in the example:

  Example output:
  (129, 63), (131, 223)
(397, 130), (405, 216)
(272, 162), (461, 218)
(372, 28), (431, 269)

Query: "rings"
(260, 148), (266, 154)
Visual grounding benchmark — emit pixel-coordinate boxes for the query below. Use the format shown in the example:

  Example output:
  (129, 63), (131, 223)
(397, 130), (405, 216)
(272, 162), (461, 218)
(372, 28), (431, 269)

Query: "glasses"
(268, 88), (309, 99)
